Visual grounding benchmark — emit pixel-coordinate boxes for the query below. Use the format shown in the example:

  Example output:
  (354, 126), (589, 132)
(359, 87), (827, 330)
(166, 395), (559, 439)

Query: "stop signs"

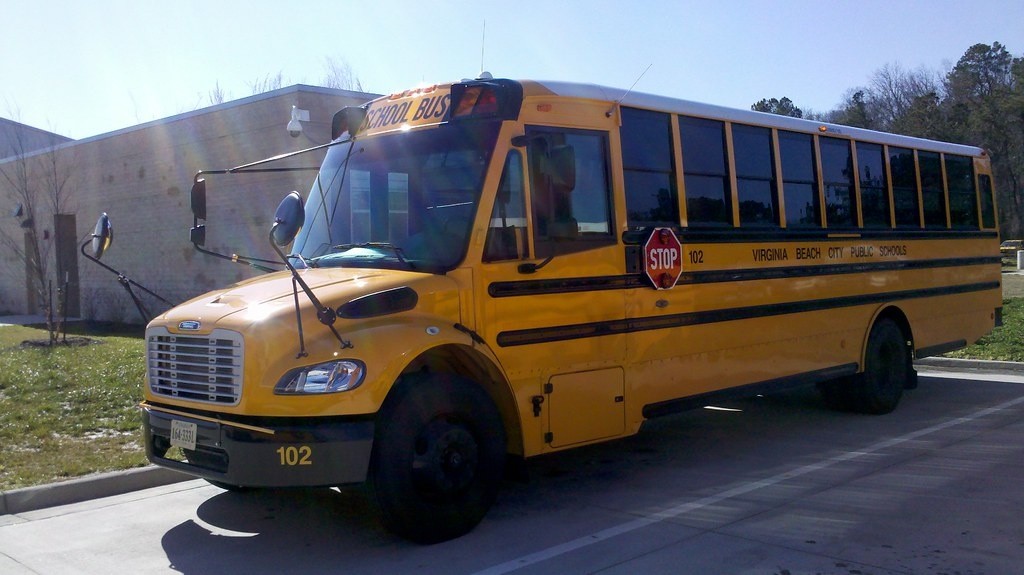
(643, 227), (683, 291)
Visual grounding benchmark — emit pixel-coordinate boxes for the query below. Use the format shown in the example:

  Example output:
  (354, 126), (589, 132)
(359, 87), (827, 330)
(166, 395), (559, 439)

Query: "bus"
(82, 70), (1005, 547)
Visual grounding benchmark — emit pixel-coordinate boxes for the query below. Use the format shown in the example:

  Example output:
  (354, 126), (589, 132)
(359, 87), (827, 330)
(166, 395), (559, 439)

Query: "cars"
(1000, 237), (1024, 256)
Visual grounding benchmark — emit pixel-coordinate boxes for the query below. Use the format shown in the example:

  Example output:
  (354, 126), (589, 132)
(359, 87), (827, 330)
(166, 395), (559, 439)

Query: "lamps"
(13, 203), (35, 228)
